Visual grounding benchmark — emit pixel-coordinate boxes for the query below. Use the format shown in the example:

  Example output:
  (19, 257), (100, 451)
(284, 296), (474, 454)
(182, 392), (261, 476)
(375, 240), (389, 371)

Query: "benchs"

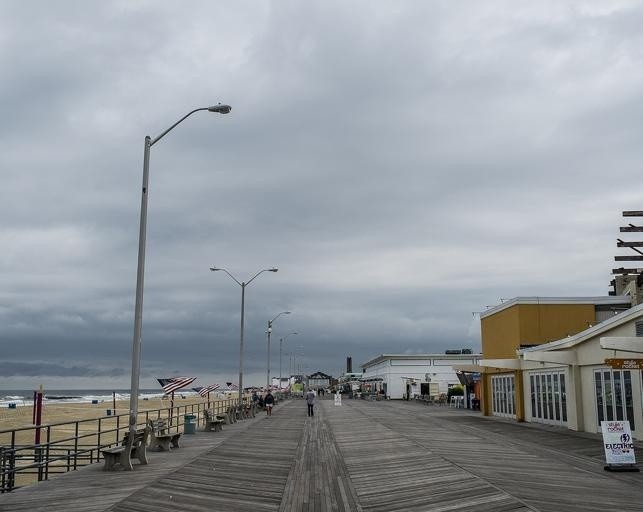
(98, 405), (237, 471)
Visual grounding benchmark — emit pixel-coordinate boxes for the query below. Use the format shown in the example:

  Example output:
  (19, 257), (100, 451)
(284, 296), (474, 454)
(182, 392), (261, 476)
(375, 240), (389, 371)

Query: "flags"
(227, 382), (238, 390)
(193, 384), (221, 396)
(156, 375), (197, 395)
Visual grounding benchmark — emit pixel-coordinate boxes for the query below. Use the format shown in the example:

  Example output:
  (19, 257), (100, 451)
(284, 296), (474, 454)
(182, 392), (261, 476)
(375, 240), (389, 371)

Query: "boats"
(44, 395), (82, 399)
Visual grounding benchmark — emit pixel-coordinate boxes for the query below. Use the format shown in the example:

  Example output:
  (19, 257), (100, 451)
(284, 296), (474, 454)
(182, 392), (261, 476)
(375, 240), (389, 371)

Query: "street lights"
(208, 266), (278, 420)
(128, 101), (234, 459)
(265, 311), (298, 399)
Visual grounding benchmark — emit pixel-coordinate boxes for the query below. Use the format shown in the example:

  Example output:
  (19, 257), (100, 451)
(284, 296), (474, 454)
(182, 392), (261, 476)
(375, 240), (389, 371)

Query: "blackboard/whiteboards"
(601, 420), (637, 464)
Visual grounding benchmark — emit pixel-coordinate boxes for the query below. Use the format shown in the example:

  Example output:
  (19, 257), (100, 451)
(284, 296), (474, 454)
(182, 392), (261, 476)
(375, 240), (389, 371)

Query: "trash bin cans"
(471, 398), (480, 410)
(184, 415), (197, 434)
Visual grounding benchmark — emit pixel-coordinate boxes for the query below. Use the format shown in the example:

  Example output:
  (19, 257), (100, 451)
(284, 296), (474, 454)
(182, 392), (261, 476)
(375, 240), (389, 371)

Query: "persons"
(322, 387), (324, 396)
(305, 389), (315, 417)
(252, 390), (275, 416)
(317, 387), (321, 396)
